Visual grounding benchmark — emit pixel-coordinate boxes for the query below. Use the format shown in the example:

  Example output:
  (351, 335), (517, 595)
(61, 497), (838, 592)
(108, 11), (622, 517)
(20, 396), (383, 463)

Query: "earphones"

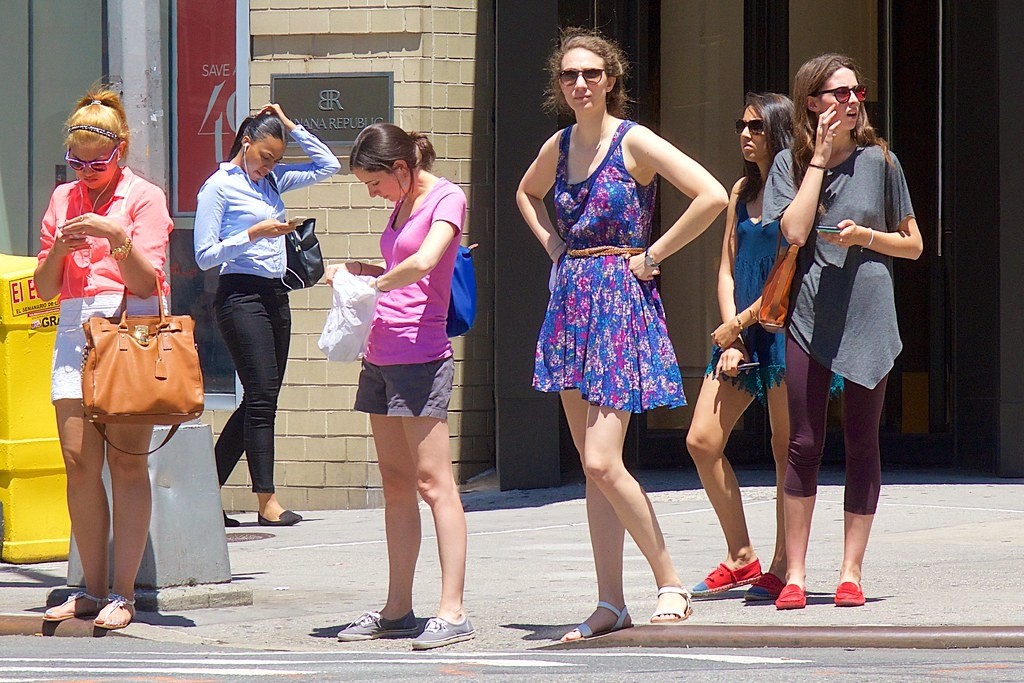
(244, 142), (249, 153)
(116, 149), (121, 160)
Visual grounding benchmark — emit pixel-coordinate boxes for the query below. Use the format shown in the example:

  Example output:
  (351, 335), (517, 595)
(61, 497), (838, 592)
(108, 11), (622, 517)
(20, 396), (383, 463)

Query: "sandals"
(93, 593), (136, 629)
(571, 601), (634, 641)
(42, 591), (111, 621)
(650, 586), (693, 624)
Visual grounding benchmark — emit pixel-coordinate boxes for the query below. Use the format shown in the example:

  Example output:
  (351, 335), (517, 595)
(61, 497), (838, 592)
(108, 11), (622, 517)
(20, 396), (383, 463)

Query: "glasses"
(735, 118), (764, 134)
(558, 68), (607, 87)
(808, 85), (866, 104)
(64, 147), (118, 173)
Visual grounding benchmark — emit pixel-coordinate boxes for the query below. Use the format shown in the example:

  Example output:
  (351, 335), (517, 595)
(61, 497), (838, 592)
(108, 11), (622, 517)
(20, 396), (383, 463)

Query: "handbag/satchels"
(445, 242), (478, 337)
(758, 243), (800, 328)
(286, 218), (324, 290)
(317, 268), (378, 363)
(81, 272), (204, 459)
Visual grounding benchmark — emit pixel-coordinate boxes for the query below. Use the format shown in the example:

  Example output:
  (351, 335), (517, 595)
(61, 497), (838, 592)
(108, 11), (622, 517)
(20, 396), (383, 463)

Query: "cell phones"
(720, 362), (760, 374)
(815, 225), (840, 234)
(59, 226), (87, 240)
(287, 216), (306, 226)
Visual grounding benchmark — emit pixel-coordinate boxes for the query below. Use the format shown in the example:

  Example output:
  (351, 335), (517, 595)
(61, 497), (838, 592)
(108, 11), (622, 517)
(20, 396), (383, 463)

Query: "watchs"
(110, 237), (132, 260)
(645, 246), (660, 268)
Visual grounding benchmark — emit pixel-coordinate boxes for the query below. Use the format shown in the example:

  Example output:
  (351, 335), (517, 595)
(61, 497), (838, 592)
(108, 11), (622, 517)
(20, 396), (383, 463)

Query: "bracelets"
(868, 228), (874, 245)
(808, 163), (825, 169)
(549, 242), (564, 257)
(354, 261), (362, 274)
(738, 314), (743, 331)
(376, 276), (390, 292)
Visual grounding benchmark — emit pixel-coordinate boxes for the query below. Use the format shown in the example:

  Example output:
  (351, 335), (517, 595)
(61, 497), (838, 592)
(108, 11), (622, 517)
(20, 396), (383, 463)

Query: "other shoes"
(257, 509), (302, 526)
(222, 509), (240, 527)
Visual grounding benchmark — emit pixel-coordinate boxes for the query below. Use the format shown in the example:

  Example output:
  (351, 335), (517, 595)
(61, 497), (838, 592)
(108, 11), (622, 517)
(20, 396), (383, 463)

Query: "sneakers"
(744, 573), (784, 600)
(834, 583), (865, 605)
(337, 610), (419, 640)
(691, 558), (762, 595)
(412, 618), (475, 649)
(775, 583), (806, 608)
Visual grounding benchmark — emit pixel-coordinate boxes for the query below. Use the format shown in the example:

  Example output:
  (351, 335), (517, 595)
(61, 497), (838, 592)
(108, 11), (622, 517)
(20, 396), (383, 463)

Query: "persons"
(327, 124), (475, 650)
(763, 54), (925, 609)
(34, 91), (174, 629)
(193, 103), (342, 528)
(517, 35), (729, 641)
(685, 92), (791, 606)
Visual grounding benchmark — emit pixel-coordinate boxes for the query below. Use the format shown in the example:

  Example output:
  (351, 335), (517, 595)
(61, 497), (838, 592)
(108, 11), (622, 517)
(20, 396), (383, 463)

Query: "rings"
(63, 240), (65, 242)
(80, 216), (83, 222)
(274, 228), (278, 232)
(70, 246), (72, 248)
(840, 238), (842, 242)
